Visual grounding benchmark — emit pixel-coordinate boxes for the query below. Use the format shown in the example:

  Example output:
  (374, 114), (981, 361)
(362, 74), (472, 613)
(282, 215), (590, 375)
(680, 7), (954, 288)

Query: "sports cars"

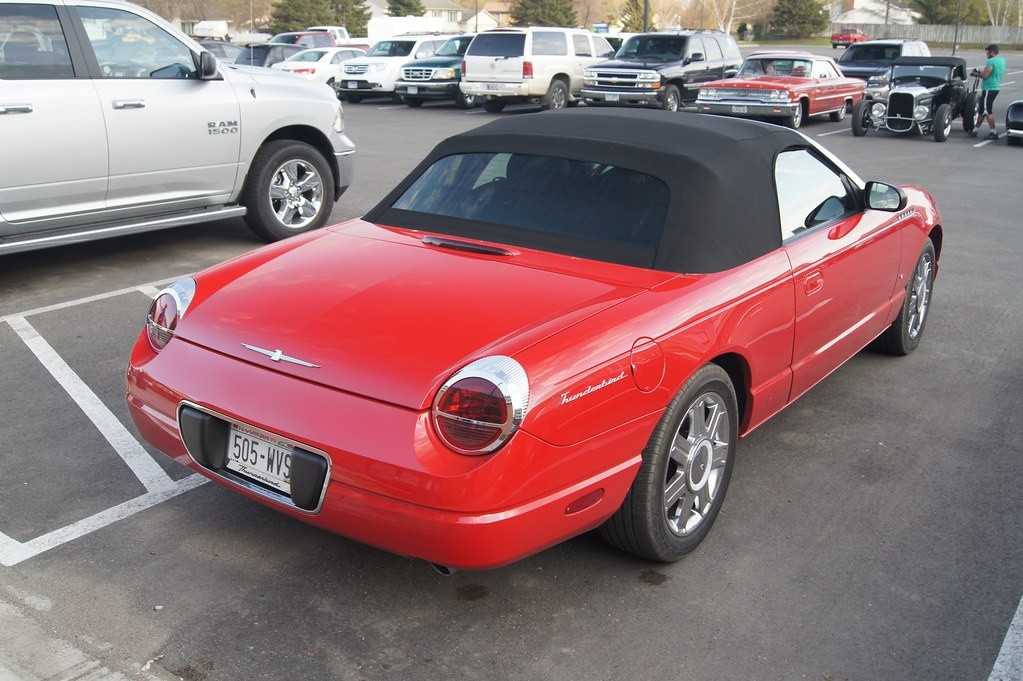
(120, 104), (946, 575)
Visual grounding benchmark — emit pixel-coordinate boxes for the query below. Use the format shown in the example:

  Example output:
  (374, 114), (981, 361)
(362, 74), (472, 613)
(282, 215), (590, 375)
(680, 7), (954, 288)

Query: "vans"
(833, 39), (931, 83)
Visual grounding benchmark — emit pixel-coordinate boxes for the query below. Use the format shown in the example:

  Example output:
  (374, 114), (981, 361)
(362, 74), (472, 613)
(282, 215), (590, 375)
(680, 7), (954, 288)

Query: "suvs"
(195, 21), (744, 146)
(0, 1), (359, 258)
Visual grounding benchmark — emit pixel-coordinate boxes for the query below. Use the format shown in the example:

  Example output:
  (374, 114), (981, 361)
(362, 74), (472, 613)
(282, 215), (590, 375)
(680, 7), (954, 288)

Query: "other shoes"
(984, 132), (998, 140)
(967, 128), (977, 137)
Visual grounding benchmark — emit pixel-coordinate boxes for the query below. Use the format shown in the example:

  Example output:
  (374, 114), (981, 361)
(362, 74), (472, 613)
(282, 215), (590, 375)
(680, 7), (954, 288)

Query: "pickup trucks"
(831, 27), (870, 50)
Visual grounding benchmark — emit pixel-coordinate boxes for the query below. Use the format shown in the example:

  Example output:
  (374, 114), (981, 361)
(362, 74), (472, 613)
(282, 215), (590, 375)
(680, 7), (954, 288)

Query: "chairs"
(464, 153), (671, 248)
(0, 31), (50, 80)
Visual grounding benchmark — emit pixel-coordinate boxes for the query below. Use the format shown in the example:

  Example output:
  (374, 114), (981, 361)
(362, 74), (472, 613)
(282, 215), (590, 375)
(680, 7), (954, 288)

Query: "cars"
(690, 50), (868, 130)
(851, 53), (983, 143)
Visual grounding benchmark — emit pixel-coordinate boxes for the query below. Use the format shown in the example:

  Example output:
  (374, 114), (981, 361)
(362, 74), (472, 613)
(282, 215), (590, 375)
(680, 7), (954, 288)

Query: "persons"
(967, 44), (1006, 140)
